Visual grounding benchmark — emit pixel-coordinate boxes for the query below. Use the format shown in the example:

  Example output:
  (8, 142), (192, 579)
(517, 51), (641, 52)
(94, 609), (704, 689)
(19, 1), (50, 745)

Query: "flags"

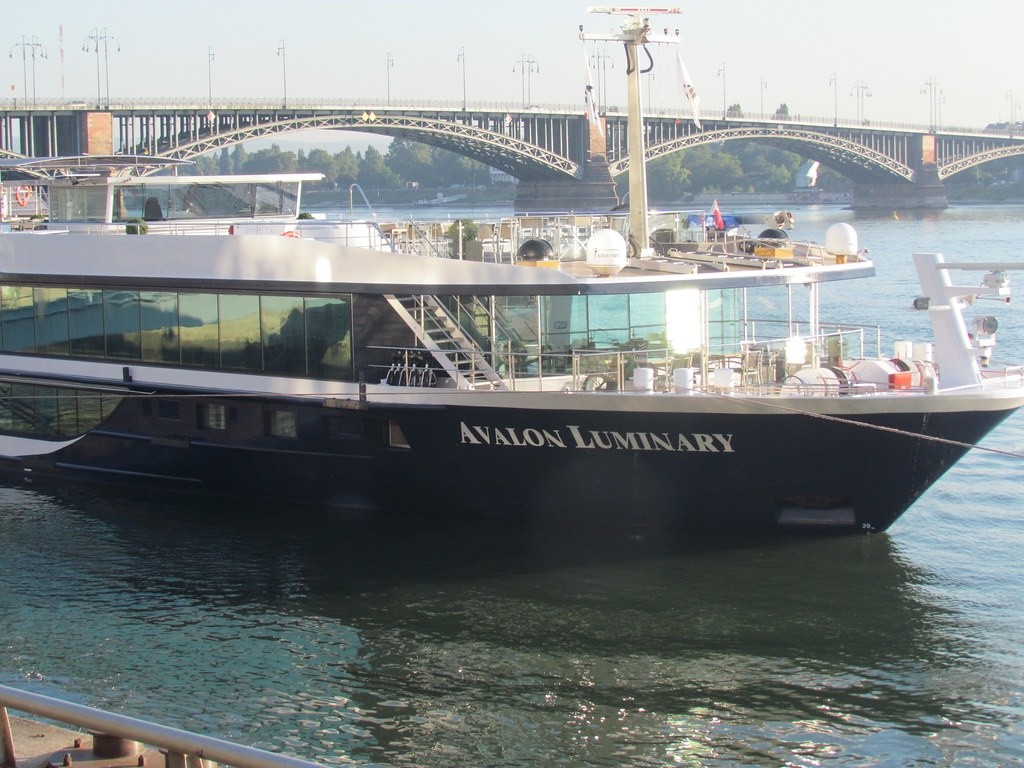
(711, 200), (724, 230)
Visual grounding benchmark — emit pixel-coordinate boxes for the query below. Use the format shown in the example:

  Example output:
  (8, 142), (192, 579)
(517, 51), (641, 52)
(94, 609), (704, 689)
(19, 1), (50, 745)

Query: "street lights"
(829, 79), (837, 127)
(277, 47), (286, 109)
(760, 78), (768, 118)
(1005, 90), (1014, 138)
(588, 40), (614, 117)
(648, 73), (655, 114)
(8, 34), (49, 108)
(387, 60), (394, 107)
(81, 27), (120, 105)
(850, 81), (873, 127)
(718, 68), (726, 120)
(208, 53), (215, 104)
(457, 49), (466, 110)
(512, 61), (540, 111)
(920, 78), (943, 130)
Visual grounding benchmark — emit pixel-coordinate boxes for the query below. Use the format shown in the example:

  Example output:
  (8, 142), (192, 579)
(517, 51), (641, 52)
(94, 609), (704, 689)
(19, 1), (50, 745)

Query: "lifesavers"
(14, 184), (31, 209)
(278, 230), (301, 239)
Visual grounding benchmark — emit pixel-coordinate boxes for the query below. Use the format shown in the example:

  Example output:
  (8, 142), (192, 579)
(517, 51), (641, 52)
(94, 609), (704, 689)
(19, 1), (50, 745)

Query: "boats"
(0, 6), (1022, 542)
